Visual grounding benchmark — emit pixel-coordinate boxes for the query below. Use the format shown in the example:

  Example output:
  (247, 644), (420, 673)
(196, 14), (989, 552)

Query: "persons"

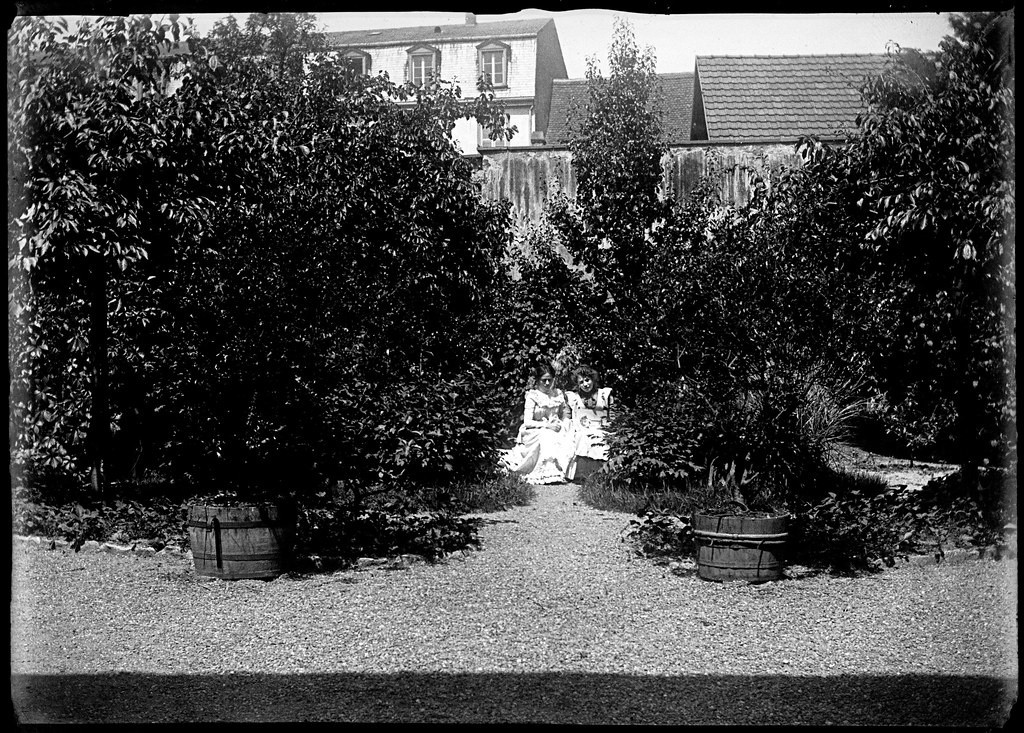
(559, 367), (615, 481)
(498, 365), (572, 487)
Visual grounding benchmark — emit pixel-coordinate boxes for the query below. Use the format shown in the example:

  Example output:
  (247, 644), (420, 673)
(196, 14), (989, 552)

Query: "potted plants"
(524, 19), (882, 581)
(8, 16), (532, 579)
